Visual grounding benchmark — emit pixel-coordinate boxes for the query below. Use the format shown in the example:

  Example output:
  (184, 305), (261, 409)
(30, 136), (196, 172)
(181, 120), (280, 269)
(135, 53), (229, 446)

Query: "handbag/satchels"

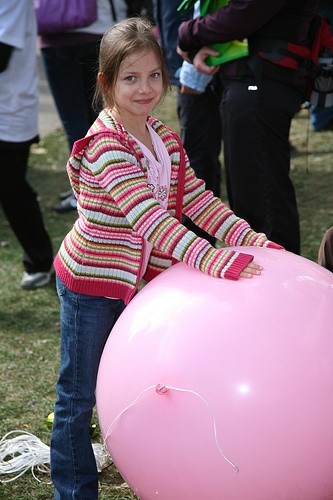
(34, 0), (98, 34)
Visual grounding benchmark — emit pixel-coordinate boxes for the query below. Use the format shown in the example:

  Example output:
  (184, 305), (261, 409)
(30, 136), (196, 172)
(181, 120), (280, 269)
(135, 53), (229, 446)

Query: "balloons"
(96, 246), (333, 500)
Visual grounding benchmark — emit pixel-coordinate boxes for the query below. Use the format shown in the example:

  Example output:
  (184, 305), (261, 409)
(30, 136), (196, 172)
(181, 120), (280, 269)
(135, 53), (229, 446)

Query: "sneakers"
(20, 263), (55, 290)
(52, 194), (77, 211)
(58, 188), (72, 200)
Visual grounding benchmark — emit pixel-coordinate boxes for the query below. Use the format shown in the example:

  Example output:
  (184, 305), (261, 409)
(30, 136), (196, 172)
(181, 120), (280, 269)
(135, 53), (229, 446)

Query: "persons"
(48, 18), (285, 500)
(174, 0), (332, 272)
(0, 0), (58, 289)
(33, 0), (125, 212)
(153, 0), (227, 246)
